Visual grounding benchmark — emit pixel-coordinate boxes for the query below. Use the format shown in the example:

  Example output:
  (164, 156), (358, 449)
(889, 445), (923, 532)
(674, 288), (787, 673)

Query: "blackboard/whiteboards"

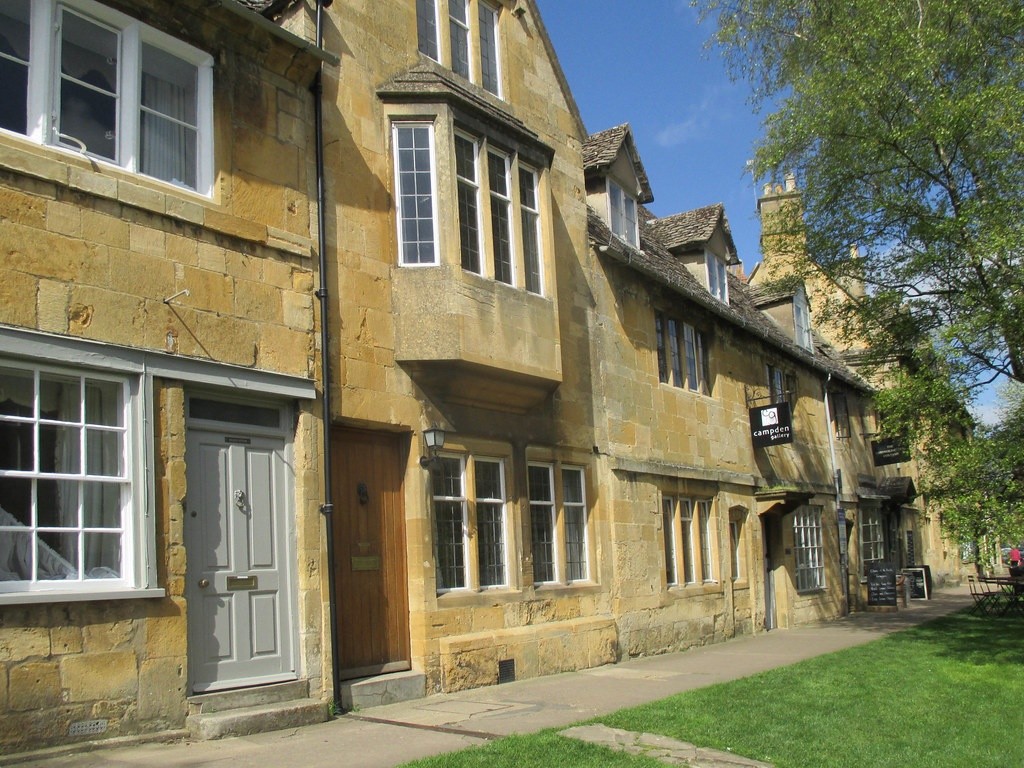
(866, 562), (898, 607)
(900, 568), (928, 600)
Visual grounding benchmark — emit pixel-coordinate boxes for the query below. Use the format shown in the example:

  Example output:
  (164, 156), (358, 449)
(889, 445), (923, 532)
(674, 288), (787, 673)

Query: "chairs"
(968, 574), (1024, 617)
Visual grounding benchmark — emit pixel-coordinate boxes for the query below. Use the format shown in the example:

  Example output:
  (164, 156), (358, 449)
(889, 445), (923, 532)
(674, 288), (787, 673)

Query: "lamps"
(419, 421), (446, 469)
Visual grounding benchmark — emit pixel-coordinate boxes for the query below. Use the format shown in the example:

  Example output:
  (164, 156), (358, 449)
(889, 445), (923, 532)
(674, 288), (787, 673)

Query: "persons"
(1009, 549), (1021, 567)
